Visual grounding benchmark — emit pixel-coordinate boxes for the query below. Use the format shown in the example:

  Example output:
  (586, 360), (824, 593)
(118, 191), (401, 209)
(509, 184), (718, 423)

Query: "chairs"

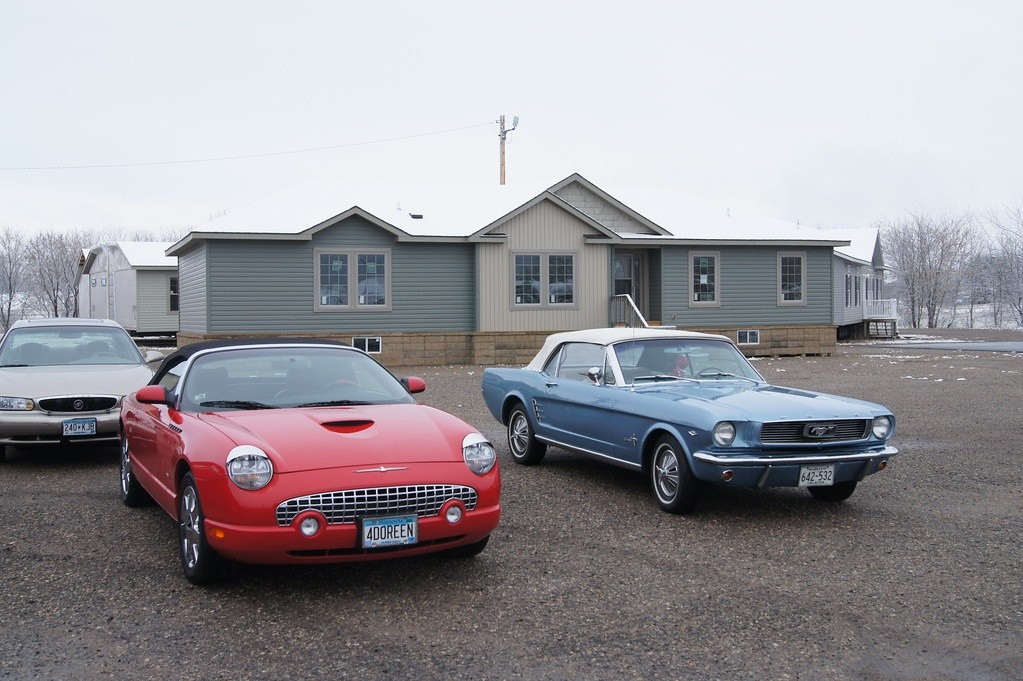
(287, 368), (341, 401)
(12, 342), (58, 362)
(84, 341), (112, 358)
(190, 367), (240, 402)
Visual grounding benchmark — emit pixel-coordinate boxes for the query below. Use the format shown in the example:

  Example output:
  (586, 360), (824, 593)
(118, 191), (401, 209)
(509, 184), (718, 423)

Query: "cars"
(118, 335), (503, 585)
(478, 328), (899, 513)
(0, 316), (157, 462)
